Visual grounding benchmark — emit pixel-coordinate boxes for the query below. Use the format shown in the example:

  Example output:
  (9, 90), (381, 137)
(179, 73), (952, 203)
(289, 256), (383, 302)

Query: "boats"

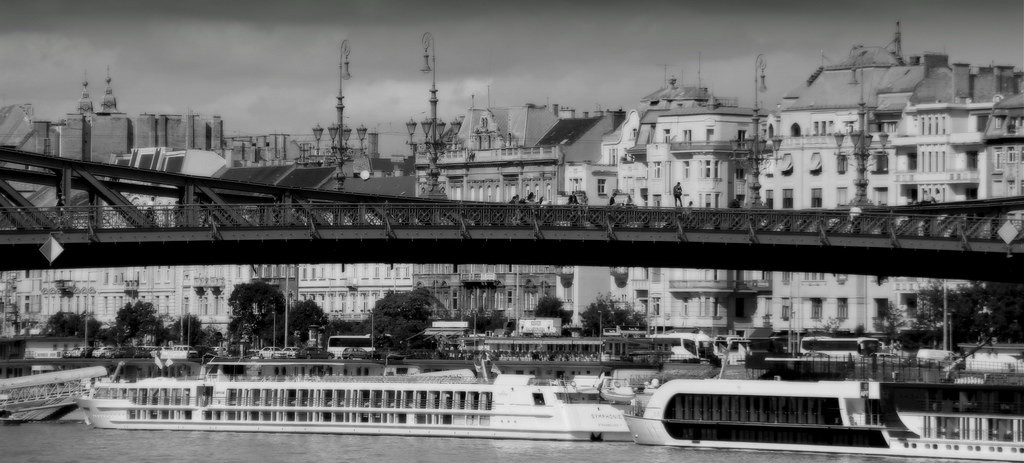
(0, 364), (108, 425)
(622, 324), (1024, 463)
(80, 368), (660, 442)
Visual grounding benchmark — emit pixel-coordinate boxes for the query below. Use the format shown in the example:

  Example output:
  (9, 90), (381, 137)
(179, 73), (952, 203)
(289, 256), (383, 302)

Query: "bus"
(801, 336), (883, 369)
(327, 333), (395, 359)
(642, 327), (752, 366)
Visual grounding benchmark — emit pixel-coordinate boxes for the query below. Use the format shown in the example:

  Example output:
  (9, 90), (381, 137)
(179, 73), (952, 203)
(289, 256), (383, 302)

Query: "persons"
(609, 193), (617, 205)
(568, 191), (580, 205)
(687, 201), (693, 206)
(625, 194), (634, 204)
(580, 192), (589, 205)
(509, 192), (544, 205)
(673, 182), (683, 207)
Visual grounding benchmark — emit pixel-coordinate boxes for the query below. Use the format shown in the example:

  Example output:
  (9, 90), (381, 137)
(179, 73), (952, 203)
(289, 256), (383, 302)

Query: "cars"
(871, 351), (908, 367)
(283, 346), (301, 358)
(259, 347), (287, 359)
(62, 344), (174, 360)
(342, 347), (370, 360)
(211, 347), (228, 358)
(295, 347), (335, 360)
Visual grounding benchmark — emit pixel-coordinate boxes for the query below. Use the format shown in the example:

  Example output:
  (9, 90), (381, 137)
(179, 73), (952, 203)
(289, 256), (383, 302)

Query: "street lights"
(334, 39), (352, 194)
(847, 43), (874, 206)
(418, 32), (450, 202)
(744, 54), (771, 210)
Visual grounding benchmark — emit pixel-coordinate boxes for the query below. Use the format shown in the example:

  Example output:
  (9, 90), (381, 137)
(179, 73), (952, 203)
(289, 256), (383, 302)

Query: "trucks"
(916, 347), (961, 371)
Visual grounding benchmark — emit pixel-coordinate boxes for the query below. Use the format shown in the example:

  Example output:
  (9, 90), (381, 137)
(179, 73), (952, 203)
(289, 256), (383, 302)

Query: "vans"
(174, 345), (198, 359)
